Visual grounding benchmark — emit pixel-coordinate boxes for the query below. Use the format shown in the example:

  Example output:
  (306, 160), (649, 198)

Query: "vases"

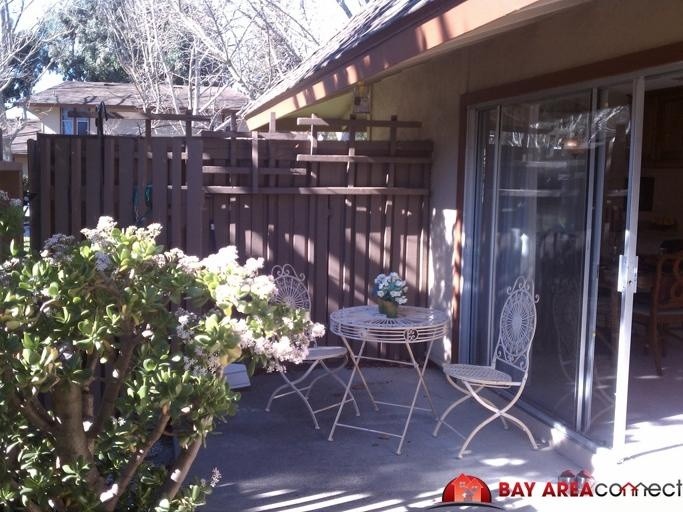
(377, 299), (387, 314)
(386, 302), (398, 318)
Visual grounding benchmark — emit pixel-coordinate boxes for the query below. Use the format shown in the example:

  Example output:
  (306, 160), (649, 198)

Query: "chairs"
(631, 251), (683, 376)
(431, 274), (539, 460)
(263, 263), (359, 431)
(557, 273), (615, 439)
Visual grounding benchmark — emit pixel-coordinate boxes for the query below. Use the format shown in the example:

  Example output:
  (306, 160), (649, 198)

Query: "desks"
(600, 272), (682, 367)
(326, 305), (450, 455)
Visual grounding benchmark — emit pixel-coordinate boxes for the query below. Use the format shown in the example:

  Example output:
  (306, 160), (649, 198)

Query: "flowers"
(385, 271), (410, 304)
(373, 271), (389, 299)
